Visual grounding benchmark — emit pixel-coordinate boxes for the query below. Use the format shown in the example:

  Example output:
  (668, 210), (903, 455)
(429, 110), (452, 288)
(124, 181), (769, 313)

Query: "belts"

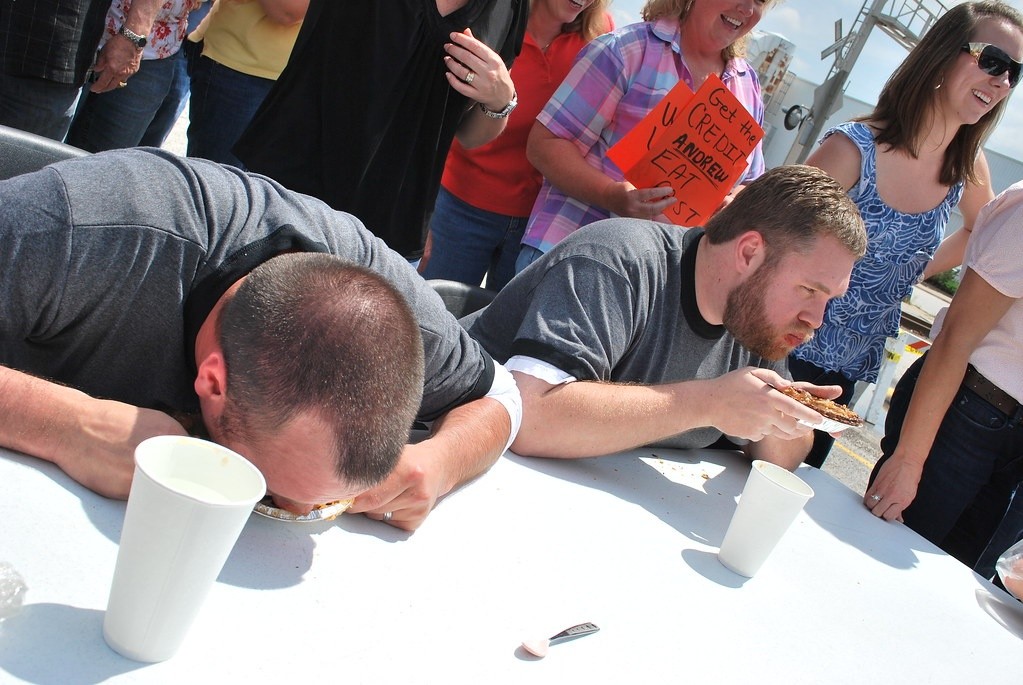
(922, 350), (1023, 426)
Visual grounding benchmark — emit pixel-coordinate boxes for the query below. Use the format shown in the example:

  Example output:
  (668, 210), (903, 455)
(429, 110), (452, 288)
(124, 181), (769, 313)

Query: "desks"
(0, 453), (1023, 685)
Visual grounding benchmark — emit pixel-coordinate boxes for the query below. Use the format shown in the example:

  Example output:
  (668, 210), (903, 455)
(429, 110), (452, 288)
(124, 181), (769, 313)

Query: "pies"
(782, 385), (862, 426)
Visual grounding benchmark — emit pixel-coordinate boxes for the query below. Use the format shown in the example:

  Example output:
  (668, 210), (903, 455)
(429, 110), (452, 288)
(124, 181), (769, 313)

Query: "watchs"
(117, 23), (147, 50)
(480, 91), (518, 119)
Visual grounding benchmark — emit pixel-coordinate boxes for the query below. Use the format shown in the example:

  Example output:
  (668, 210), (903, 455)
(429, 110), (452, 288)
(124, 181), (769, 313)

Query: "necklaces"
(542, 42), (551, 49)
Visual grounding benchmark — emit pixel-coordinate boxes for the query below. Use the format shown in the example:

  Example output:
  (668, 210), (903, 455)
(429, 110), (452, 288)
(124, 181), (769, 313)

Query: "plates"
(796, 411), (863, 433)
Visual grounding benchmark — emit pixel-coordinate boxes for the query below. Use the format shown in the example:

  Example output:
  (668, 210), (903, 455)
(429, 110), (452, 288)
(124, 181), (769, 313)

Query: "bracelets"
(917, 274), (925, 284)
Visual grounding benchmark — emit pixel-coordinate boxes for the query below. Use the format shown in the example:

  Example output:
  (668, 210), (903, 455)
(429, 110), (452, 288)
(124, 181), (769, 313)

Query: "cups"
(717, 459), (814, 578)
(102, 435), (267, 663)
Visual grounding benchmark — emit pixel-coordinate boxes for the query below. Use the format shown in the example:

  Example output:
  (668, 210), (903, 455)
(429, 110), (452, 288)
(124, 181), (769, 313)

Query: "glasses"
(960, 42), (1023, 89)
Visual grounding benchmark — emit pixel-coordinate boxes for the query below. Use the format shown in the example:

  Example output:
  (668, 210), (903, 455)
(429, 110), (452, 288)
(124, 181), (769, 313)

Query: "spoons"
(522, 621), (600, 659)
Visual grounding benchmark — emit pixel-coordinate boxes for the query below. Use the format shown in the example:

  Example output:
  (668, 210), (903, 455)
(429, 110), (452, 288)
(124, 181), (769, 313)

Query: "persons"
(863, 177), (1023, 606)
(455, 163), (868, 478)
(0, 0), (789, 292)
(796, 0), (1023, 467)
(0, 146), (524, 530)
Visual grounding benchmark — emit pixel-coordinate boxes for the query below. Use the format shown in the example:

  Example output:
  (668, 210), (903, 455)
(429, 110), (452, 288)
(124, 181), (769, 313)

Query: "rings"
(869, 494), (883, 502)
(382, 512), (393, 522)
(120, 81), (127, 88)
(465, 71), (475, 85)
